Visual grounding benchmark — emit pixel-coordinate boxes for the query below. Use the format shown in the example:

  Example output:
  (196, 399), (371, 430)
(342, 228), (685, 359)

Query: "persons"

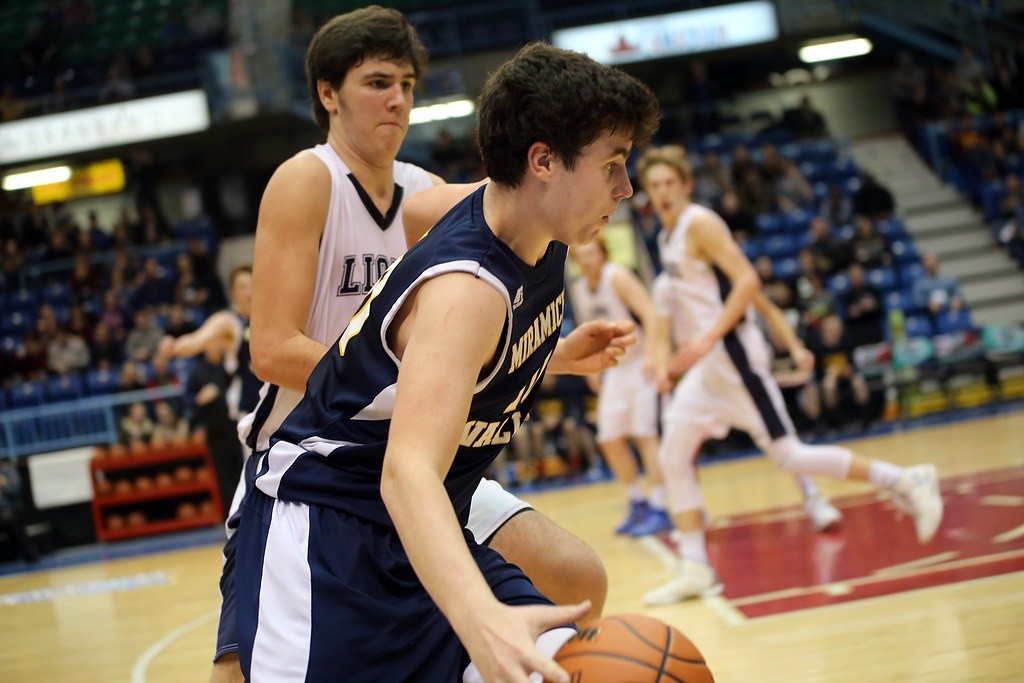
(224, 4), (610, 624)
(3, 0), (1023, 455)
(212, 42), (661, 683)
(559, 237), (673, 544)
(631, 141), (945, 609)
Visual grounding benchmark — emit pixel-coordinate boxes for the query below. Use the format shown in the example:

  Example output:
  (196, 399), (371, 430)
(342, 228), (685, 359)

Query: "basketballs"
(541, 612), (714, 683)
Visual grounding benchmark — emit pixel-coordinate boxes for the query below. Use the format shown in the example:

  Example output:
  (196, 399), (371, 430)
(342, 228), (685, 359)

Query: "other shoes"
(824, 512), (827, 513)
(892, 462), (944, 544)
(643, 561), (724, 604)
(630, 508), (675, 537)
(614, 499), (653, 534)
(803, 495), (843, 532)
